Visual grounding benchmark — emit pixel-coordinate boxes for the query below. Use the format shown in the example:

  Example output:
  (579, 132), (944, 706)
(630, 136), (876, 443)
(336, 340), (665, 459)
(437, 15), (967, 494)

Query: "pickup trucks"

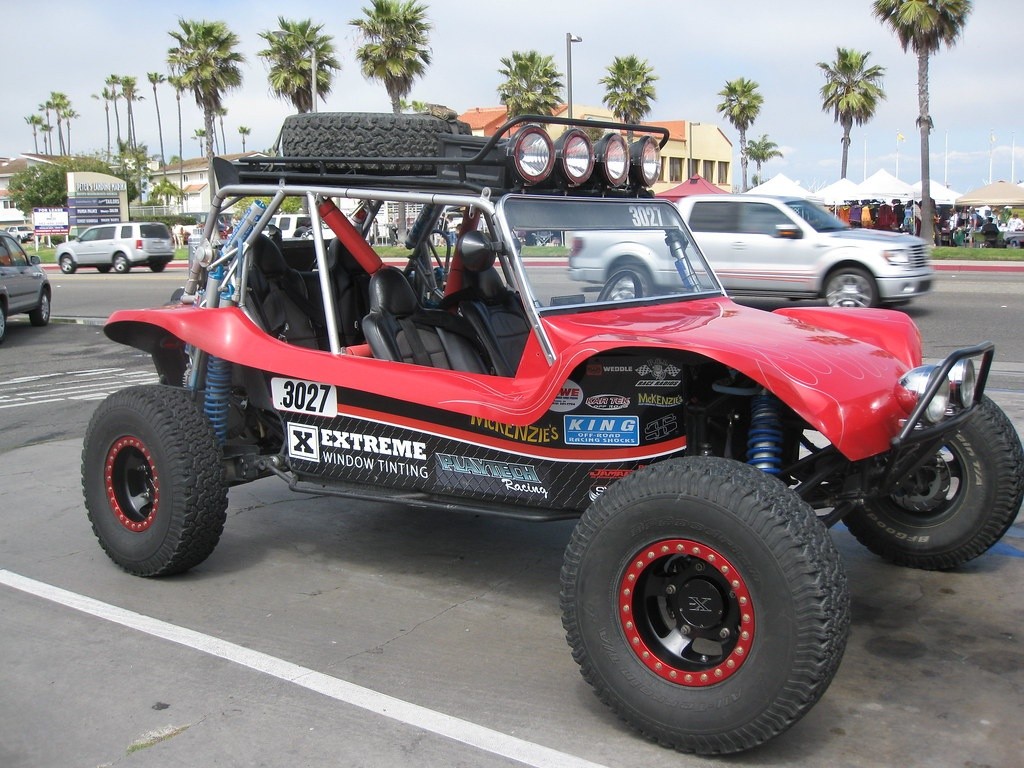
(261, 213), (339, 241)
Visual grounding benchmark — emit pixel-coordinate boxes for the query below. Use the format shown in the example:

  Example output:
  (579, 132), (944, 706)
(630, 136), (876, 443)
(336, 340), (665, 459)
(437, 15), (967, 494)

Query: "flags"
(897, 133), (904, 142)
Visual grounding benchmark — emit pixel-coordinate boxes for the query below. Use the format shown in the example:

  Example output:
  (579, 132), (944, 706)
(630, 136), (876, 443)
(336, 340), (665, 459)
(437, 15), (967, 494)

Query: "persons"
(1006, 211), (1024, 231)
(982, 217), (999, 247)
(436, 212), (527, 260)
(966, 206), (979, 247)
(370, 218), (379, 245)
(946, 207), (959, 247)
(934, 210), (942, 236)
(385, 216), (397, 246)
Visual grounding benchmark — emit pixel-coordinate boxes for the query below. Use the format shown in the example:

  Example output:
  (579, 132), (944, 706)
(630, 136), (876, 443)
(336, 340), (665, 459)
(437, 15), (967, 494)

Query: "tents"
(652, 172), (734, 204)
(814, 168), (1024, 209)
(739, 173), (825, 208)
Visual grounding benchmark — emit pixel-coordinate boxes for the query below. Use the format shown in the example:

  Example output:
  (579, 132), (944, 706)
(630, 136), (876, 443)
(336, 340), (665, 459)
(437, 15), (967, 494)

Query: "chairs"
(360, 267), (488, 375)
(982, 230), (999, 248)
(247, 234), (320, 350)
(438, 263), (531, 377)
(328, 237), (370, 346)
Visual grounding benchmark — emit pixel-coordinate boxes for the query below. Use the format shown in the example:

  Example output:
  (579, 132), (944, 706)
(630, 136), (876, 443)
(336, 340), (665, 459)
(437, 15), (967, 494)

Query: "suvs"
(54, 221), (176, 274)
(79, 103), (1018, 757)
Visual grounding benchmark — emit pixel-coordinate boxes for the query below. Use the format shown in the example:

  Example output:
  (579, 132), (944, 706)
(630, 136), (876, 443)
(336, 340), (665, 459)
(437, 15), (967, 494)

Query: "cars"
(558, 192), (936, 311)
(0, 228), (52, 341)
(6, 225), (35, 244)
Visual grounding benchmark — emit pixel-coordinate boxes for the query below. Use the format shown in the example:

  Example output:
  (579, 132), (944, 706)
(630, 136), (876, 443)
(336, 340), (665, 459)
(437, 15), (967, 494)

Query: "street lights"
(271, 29), (318, 115)
(566, 31), (583, 130)
